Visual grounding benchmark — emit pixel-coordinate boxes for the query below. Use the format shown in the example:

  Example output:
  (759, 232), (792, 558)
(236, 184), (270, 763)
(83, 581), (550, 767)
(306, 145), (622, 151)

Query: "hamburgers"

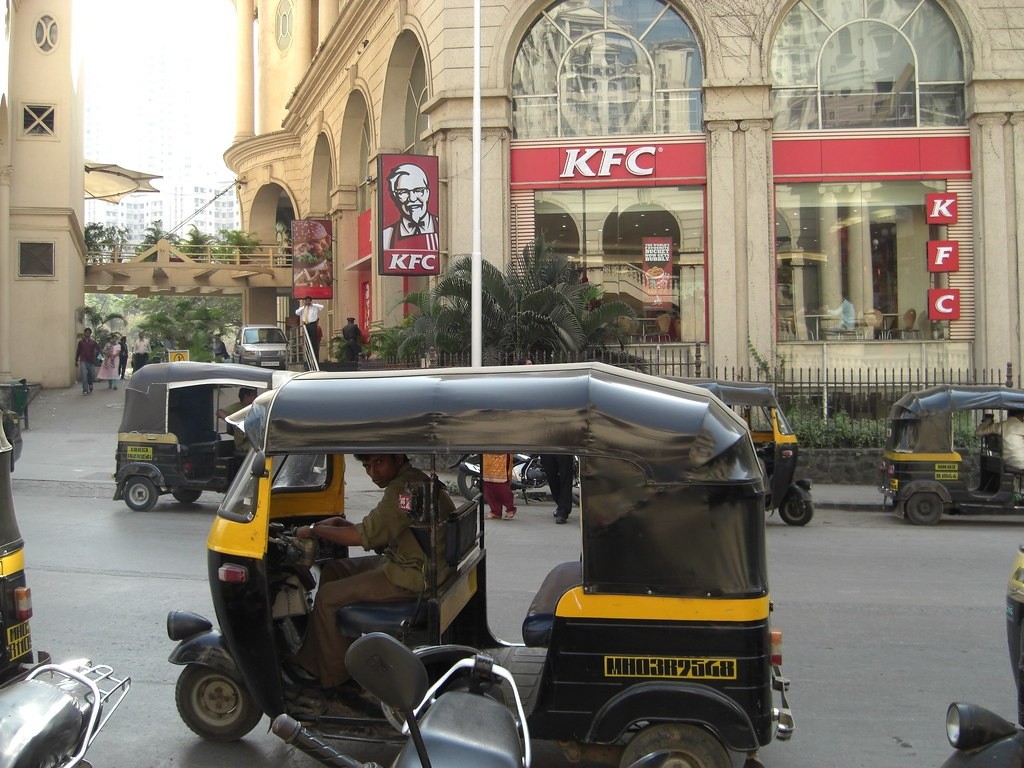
(292, 220), (332, 287)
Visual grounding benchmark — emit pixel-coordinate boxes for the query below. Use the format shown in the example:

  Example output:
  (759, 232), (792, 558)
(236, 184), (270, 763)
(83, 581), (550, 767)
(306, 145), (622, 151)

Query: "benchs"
(335, 502), (478, 643)
(983, 454), (1024, 478)
(522, 561), (582, 648)
(161, 428), (222, 454)
(960, 456), (974, 471)
(215, 438), (241, 464)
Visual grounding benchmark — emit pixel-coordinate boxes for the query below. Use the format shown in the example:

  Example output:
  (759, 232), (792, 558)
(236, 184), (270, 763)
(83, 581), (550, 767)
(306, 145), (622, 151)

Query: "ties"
(305, 306), (309, 324)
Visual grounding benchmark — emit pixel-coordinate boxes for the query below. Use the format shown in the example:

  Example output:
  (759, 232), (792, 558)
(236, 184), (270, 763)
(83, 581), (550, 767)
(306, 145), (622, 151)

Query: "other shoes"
(505, 508), (515, 519)
(282, 661), (317, 689)
(104, 364), (108, 368)
(484, 512), (502, 520)
(121, 377), (125, 379)
(556, 516), (567, 524)
(114, 387), (117, 390)
(109, 385), (112, 389)
(83, 391), (88, 396)
(553, 510), (561, 517)
(89, 384), (93, 391)
(110, 363), (115, 368)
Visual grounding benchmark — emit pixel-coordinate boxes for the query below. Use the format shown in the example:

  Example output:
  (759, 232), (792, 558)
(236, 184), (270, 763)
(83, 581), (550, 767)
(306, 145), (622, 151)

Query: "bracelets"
(310, 523), (318, 536)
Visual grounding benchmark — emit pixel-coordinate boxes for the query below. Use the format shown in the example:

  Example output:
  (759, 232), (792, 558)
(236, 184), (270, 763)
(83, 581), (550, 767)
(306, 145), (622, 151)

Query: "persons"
(206, 332), (229, 363)
(975, 408), (1024, 470)
(481, 453), (517, 519)
(117, 337), (128, 379)
(74, 328), (105, 395)
(281, 453), (455, 696)
(342, 317), (361, 361)
(819, 292), (855, 339)
(96, 335), (121, 390)
(316, 318), (323, 351)
(295, 296), (324, 361)
(539, 454), (574, 524)
(133, 332), (152, 371)
(216, 388), (259, 452)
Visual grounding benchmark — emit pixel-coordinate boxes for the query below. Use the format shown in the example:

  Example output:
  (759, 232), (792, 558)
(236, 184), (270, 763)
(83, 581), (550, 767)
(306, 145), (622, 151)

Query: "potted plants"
(78, 307), (127, 383)
(101, 226), (133, 263)
(135, 218), (263, 263)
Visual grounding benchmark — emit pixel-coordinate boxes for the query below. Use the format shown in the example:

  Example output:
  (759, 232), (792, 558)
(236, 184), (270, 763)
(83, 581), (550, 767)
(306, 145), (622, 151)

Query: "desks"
(804, 314), (832, 341)
(636, 317), (658, 342)
(882, 313), (904, 339)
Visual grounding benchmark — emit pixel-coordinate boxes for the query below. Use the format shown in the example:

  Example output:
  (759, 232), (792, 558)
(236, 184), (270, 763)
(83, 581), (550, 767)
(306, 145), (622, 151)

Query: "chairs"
(616, 314), (681, 342)
(826, 308), (926, 339)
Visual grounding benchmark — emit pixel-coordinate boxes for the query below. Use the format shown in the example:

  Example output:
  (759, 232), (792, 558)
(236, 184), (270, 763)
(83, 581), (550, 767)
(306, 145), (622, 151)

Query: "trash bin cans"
(11, 380), (30, 414)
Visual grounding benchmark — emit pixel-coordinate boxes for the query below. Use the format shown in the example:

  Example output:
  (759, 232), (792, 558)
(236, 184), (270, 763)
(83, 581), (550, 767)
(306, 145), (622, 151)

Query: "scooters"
(271, 633), (533, 768)
(0, 657), (131, 768)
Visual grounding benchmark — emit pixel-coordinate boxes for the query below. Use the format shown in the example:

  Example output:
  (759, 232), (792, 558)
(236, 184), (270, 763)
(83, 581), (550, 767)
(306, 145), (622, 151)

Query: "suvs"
(234, 326), (291, 371)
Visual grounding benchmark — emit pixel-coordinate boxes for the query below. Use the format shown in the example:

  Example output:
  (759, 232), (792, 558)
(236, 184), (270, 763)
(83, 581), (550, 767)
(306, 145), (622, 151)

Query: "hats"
(347, 318), (355, 321)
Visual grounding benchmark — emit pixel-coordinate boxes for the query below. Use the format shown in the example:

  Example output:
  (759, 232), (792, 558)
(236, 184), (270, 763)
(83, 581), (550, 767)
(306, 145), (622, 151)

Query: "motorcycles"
(167, 361), (796, 768)
(0, 407), (35, 683)
(447, 453), (581, 507)
(938, 543), (1023, 768)
(655, 373), (814, 525)
(111, 360), (326, 514)
(876, 384), (1024, 526)
